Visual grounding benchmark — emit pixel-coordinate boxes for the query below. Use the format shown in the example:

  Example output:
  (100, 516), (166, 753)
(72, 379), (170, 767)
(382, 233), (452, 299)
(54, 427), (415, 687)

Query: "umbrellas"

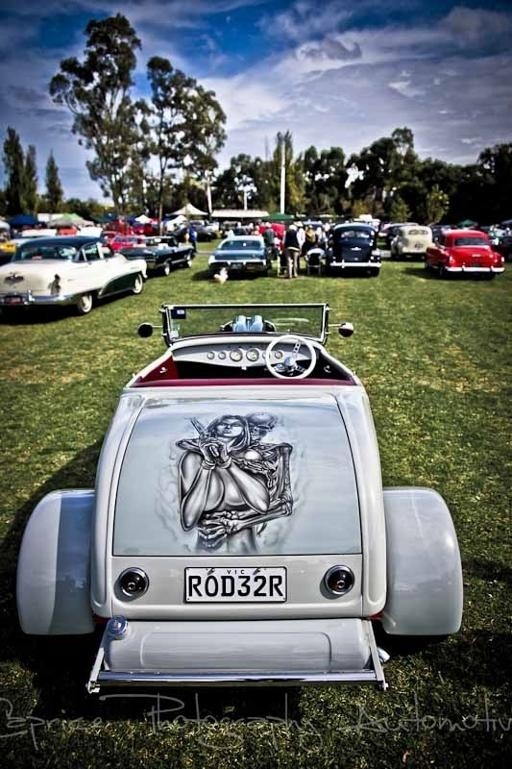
(134, 213), (154, 226)
(169, 203), (208, 222)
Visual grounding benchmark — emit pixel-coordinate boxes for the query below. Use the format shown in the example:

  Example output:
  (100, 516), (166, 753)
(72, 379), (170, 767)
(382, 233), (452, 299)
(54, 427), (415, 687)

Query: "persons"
(178, 413), (270, 557)
(252, 219), (326, 278)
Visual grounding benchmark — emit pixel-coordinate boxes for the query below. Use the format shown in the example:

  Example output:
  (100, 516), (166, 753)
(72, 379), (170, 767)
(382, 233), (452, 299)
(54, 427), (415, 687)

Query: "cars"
(0, 220), (197, 314)
(325, 222), (382, 276)
(15, 303), (463, 694)
(386, 218), (512, 280)
(208, 235), (272, 278)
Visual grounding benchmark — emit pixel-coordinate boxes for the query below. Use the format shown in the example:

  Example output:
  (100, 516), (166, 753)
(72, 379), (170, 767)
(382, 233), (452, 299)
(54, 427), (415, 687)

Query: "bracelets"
(216, 457), (233, 469)
(201, 459), (216, 470)
